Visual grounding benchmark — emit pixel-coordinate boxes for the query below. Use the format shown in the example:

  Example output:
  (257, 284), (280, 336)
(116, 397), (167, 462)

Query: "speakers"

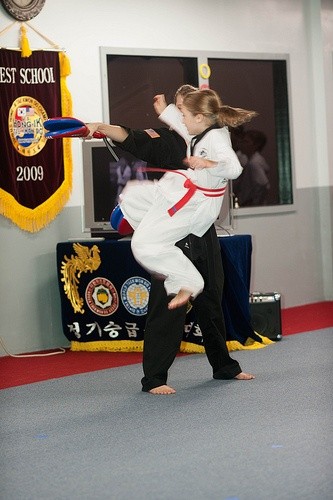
(248, 290), (282, 341)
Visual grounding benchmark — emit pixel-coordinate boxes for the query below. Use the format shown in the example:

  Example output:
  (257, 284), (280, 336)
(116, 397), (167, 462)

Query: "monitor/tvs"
(82, 141), (236, 237)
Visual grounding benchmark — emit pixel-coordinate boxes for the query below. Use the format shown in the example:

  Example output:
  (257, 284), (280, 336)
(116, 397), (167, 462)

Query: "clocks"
(1, 0), (46, 22)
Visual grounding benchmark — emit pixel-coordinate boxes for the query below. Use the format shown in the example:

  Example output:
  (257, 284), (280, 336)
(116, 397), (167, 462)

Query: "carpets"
(1, 298), (333, 389)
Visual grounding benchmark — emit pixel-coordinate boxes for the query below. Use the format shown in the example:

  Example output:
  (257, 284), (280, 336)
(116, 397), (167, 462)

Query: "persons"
(226, 123), (275, 207)
(116, 156), (147, 202)
(84, 84), (254, 395)
(120, 89), (258, 308)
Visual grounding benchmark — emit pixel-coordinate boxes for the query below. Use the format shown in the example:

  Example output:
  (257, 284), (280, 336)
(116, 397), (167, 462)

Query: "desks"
(55, 235), (253, 351)
